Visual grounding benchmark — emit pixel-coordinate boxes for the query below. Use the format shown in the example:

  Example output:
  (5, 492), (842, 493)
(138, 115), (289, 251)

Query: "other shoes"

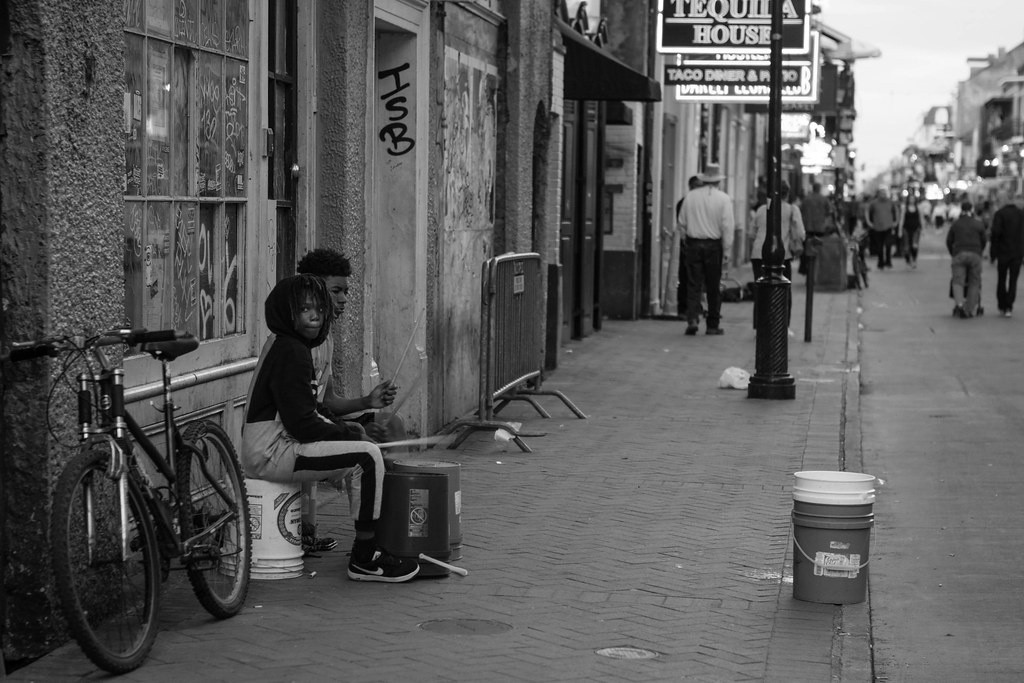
(1000, 308), (1012, 316)
(705, 328), (724, 335)
(685, 319), (698, 336)
(953, 306), (973, 318)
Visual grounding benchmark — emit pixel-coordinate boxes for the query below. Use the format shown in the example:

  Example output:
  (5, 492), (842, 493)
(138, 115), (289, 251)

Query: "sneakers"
(347, 548), (420, 582)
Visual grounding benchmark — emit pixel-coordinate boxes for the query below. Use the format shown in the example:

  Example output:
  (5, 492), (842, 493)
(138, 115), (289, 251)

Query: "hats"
(697, 163), (727, 182)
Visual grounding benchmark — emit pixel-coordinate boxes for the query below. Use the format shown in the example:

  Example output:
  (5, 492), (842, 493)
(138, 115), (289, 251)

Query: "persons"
(676, 164), (735, 337)
(746, 171), (1024, 329)
(241, 245), (421, 583)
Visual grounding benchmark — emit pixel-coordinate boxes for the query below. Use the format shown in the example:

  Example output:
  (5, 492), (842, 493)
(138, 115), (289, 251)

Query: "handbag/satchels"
(789, 204), (804, 257)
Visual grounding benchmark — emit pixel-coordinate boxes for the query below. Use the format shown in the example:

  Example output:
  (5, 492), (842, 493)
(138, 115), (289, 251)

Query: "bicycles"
(8, 326), (254, 674)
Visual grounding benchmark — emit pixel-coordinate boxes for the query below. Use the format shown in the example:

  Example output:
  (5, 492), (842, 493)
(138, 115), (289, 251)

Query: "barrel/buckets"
(388, 459), (464, 561)
(791, 471), (875, 605)
(376, 473), (468, 579)
(218, 473), (317, 580)
